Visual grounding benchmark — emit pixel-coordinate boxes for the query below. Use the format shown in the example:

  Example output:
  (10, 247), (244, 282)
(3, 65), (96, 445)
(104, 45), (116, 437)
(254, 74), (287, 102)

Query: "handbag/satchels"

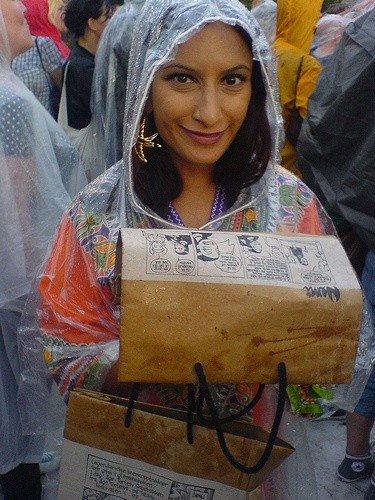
(287, 55), (304, 146)
(58, 387), (295, 499)
(58, 60), (94, 182)
(119, 227), (364, 474)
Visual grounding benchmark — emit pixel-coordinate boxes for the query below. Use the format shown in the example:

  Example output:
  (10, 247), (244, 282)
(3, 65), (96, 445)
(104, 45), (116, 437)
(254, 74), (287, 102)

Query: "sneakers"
(337, 451), (375, 483)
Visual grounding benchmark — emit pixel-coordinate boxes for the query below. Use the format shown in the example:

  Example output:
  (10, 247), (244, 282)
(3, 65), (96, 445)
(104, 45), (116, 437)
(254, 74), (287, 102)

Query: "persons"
(250, 0), (375, 182)
(16, 0), (374, 500)
(298, 5), (375, 500)
(0, 0), (80, 500)
(11, 1), (124, 131)
